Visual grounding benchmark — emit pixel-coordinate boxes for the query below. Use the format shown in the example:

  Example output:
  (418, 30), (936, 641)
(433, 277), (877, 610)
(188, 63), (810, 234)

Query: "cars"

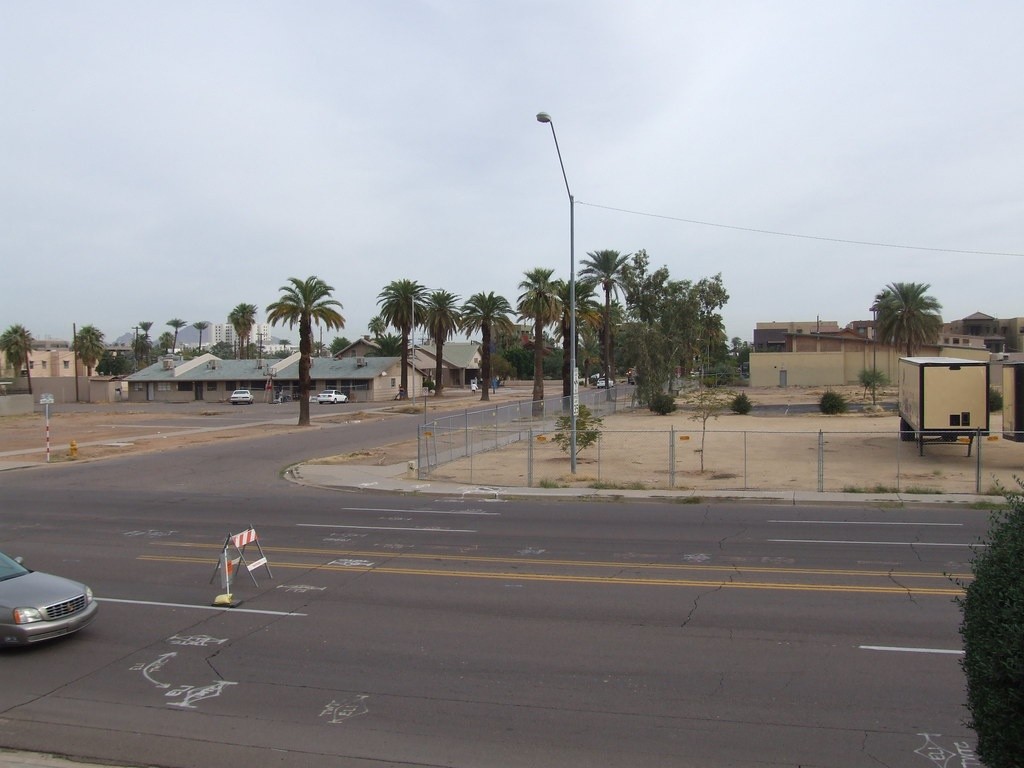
(0, 551), (98, 649)
(317, 389), (348, 404)
(230, 389), (253, 405)
(597, 377), (614, 389)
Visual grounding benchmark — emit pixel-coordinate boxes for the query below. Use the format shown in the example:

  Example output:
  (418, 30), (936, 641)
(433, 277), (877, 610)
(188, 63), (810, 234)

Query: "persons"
(471, 382), (478, 395)
(400, 385), (405, 400)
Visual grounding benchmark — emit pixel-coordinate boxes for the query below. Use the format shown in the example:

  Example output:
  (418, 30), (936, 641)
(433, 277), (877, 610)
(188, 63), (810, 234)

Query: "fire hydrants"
(70, 439), (78, 461)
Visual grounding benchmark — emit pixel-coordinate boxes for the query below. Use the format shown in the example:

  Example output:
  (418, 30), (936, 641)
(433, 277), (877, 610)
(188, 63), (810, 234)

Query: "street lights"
(537, 112), (577, 476)
